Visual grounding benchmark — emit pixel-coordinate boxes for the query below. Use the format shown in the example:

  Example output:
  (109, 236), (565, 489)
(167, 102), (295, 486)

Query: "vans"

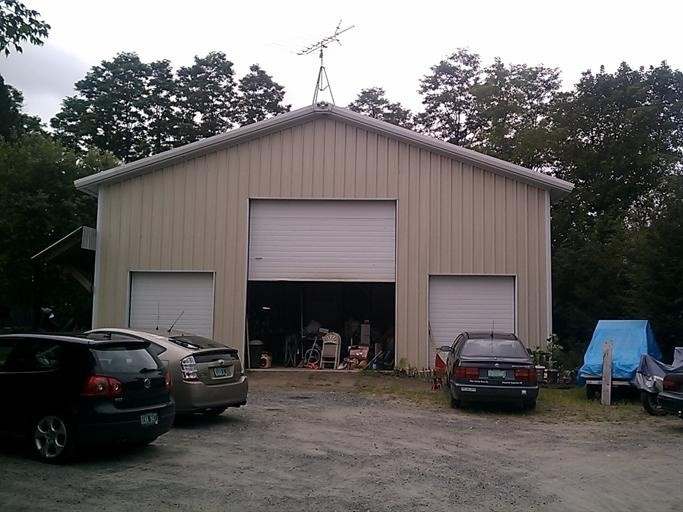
(43, 309), (247, 426)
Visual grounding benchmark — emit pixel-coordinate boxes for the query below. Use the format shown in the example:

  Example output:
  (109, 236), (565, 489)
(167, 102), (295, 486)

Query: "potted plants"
(535, 333), (571, 384)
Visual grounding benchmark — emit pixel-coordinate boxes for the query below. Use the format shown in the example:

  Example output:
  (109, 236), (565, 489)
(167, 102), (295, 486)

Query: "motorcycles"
(634, 347), (683, 416)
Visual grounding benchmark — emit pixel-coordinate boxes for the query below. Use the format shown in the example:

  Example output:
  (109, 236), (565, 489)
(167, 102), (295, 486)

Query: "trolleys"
(585, 318), (652, 402)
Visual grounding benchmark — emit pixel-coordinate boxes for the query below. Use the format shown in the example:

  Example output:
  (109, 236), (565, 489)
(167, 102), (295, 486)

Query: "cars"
(0, 329), (177, 461)
(657, 366), (683, 419)
(439, 325), (541, 413)
